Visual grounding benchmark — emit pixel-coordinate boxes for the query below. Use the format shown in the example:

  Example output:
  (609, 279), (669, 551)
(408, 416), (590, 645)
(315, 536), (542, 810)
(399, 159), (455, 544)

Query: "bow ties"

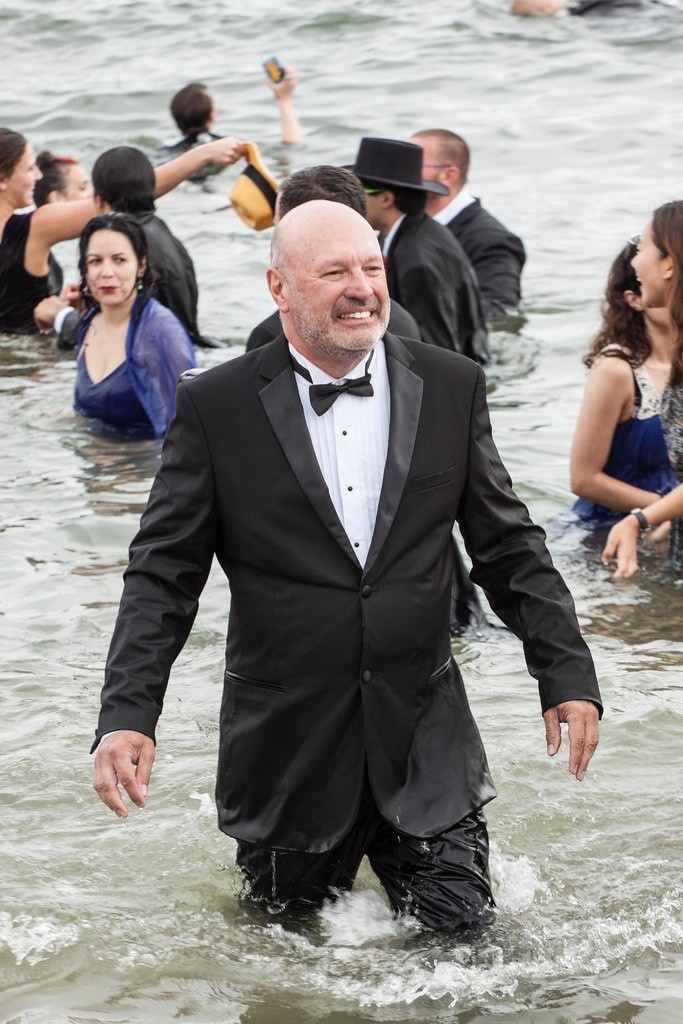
(289, 349), (378, 418)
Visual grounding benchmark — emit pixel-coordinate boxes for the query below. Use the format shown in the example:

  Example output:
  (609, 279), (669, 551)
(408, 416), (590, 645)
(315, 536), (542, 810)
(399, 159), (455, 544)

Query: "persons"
(571, 200), (683, 581)
(90, 199), (603, 941)
(0, 58), (528, 447)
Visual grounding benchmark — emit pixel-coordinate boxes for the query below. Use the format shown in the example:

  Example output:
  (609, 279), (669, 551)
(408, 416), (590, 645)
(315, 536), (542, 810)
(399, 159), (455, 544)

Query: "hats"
(230, 143), (282, 230)
(340, 137), (450, 195)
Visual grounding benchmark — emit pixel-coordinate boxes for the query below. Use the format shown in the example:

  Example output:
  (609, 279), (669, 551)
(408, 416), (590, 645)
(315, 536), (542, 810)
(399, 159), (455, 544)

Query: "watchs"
(630, 508), (649, 530)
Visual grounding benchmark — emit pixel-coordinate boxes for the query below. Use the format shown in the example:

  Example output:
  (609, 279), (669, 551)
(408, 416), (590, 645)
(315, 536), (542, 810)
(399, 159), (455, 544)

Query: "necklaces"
(644, 357), (671, 371)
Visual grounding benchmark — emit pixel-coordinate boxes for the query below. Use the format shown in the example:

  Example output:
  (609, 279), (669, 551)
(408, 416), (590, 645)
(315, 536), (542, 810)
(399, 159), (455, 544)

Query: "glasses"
(624, 233), (642, 263)
